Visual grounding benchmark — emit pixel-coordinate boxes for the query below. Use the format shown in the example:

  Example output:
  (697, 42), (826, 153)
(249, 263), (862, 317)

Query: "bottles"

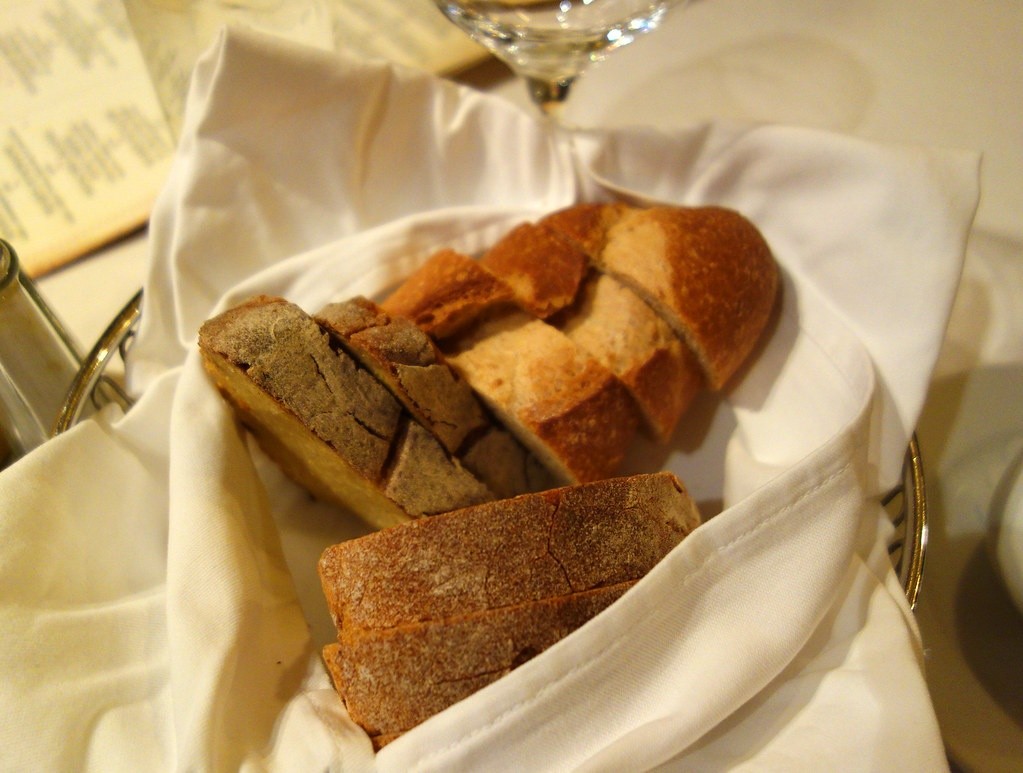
(0, 240), (127, 473)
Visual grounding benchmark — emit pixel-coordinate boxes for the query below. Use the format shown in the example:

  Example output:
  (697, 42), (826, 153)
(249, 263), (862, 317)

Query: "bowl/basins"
(50, 285), (927, 618)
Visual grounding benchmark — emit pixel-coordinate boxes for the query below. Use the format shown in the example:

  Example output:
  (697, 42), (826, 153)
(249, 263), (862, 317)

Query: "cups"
(432, 0), (672, 103)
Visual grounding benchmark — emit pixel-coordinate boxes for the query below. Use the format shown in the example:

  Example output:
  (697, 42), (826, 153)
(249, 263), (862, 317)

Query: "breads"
(197, 195), (786, 755)
